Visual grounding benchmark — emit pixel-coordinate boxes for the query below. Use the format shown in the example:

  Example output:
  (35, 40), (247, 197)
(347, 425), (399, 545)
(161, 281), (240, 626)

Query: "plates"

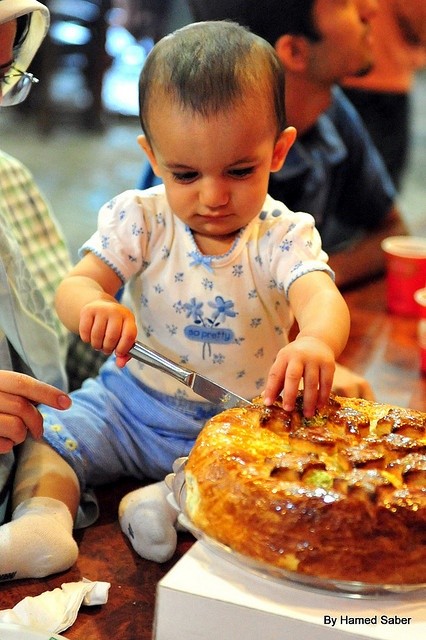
(164, 455), (426, 604)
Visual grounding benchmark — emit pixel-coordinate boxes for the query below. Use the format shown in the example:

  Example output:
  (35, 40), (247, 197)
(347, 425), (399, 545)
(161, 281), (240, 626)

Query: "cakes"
(182, 393), (426, 586)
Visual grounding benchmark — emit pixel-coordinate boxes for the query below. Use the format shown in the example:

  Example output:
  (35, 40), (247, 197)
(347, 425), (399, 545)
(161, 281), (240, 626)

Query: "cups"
(379, 234), (426, 319)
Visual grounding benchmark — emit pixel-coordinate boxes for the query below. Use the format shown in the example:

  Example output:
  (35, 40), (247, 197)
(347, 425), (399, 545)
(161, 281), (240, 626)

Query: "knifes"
(128, 339), (256, 410)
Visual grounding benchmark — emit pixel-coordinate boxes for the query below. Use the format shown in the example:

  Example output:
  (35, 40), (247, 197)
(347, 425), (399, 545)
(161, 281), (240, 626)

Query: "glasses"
(0, 66), (41, 108)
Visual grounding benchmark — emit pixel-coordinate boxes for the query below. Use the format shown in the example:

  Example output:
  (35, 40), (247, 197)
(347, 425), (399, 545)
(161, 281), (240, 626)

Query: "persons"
(0, 19), (352, 585)
(0, 0), (106, 526)
(134, 2), (411, 288)
(340, 0), (426, 193)
